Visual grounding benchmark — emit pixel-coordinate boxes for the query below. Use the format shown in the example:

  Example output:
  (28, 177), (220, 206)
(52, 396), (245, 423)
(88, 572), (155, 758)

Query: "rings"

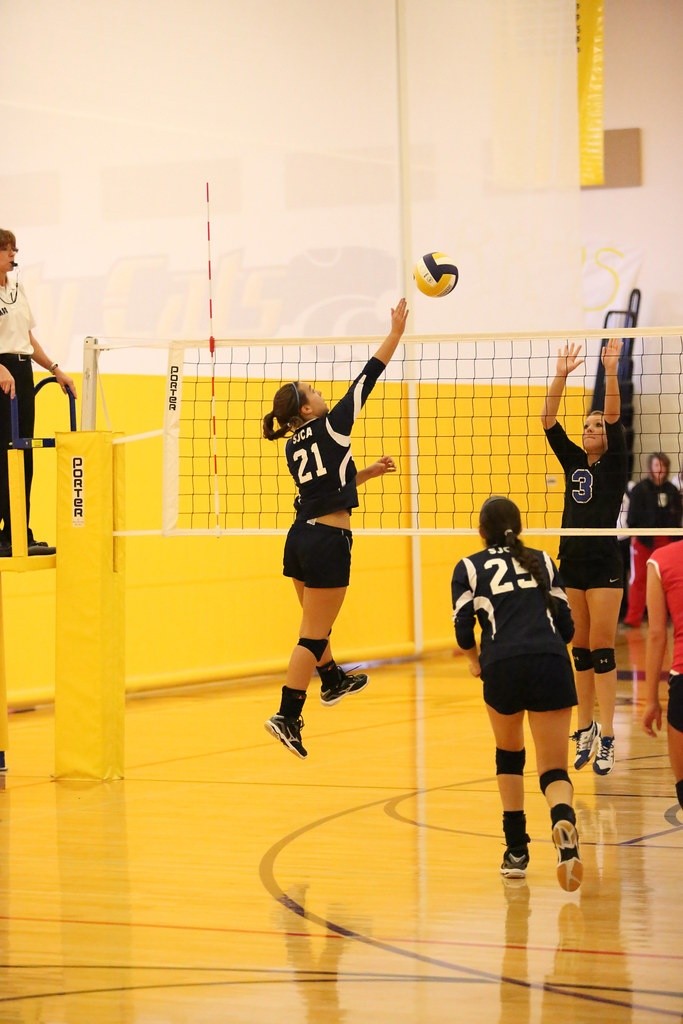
(62, 387), (65, 389)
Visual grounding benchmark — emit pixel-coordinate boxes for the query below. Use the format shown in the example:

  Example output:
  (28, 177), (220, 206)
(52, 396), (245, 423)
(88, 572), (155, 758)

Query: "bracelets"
(49, 363), (58, 372)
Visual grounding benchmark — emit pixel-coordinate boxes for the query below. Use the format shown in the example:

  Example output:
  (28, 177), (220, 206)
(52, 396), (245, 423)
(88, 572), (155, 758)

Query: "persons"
(450, 497), (584, 892)
(0, 228), (78, 559)
(618, 451), (683, 630)
(541, 334), (635, 777)
(641, 524), (683, 809)
(263, 298), (409, 760)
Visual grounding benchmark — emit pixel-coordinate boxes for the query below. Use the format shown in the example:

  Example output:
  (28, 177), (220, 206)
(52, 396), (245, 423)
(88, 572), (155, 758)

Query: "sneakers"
(550, 816), (584, 893)
(264, 712), (308, 759)
(592, 731), (615, 776)
(498, 842), (529, 879)
(567, 719), (602, 771)
(319, 664), (370, 707)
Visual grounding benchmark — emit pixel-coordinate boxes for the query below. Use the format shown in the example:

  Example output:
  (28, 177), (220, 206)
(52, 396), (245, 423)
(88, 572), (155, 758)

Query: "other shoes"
(28, 539), (49, 550)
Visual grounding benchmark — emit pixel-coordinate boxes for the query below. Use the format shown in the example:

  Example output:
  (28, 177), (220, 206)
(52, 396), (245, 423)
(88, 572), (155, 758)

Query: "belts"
(0, 353), (33, 361)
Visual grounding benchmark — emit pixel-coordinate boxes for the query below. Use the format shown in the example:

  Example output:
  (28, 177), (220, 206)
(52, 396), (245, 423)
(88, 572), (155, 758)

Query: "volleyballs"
(413, 252), (460, 298)
